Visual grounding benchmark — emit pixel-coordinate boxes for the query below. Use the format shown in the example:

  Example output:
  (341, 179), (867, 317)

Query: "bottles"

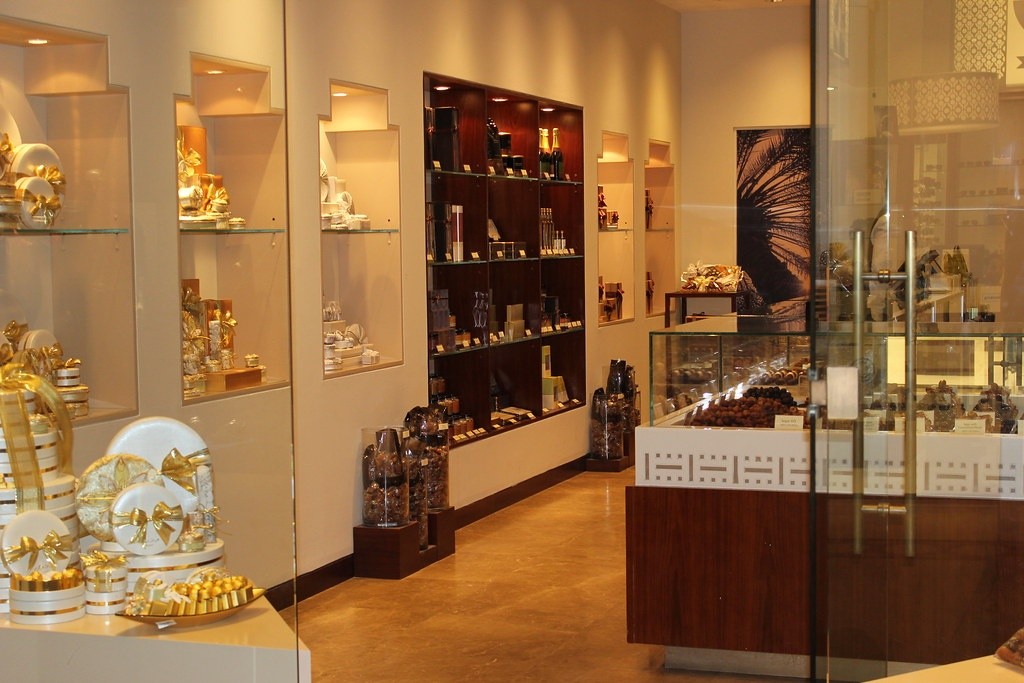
(539, 127), (563, 180)
(541, 208), (566, 252)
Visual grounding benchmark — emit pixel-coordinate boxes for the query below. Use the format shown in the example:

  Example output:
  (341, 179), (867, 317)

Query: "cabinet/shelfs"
(626, 387), (1024, 683)
(662, 291), (761, 403)
(423, 70), (587, 452)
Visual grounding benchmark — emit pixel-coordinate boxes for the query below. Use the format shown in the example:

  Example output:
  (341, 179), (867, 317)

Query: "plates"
(114, 587), (267, 628)
